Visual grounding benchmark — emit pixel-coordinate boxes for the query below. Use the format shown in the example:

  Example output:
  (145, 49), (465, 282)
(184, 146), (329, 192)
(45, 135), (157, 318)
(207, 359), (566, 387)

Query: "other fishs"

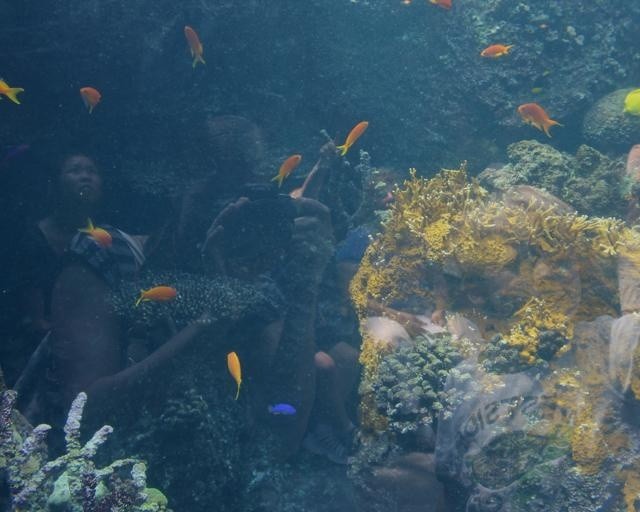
(480, 43), (515, 57)
(79, 86), (101, 115)
(429, 0), (453, 11)
(517, 102), (565, 139)
(135, 285), (182, 310)
(75, 217), (113, 249)
(623, 88), (640, 115)
(270, 154), (302, 189)
(184, 24), (208, 70)
(314, 350), (336, 370)
(225, 349), (245, 401)
(0, 79), (24, 105)
(266, 401), (298, 416)
(336, 120), (369, 157)
